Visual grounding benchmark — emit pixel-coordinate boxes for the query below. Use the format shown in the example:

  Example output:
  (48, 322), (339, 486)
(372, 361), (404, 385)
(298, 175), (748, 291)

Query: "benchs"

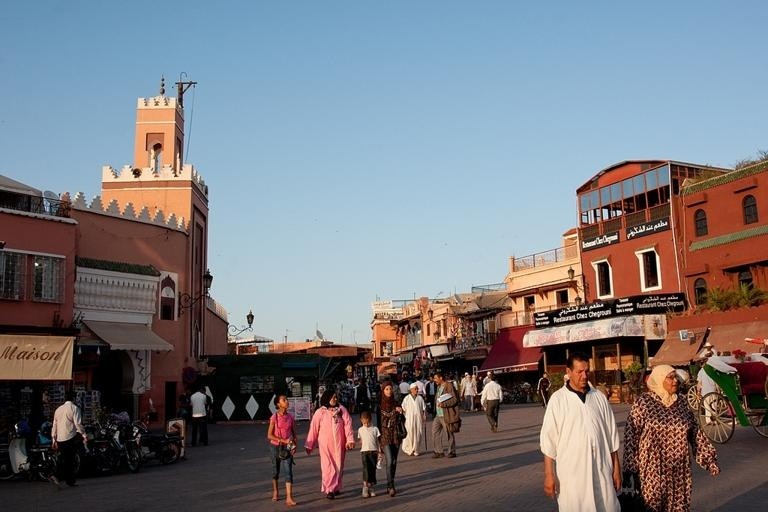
(726, 361), (767, 396)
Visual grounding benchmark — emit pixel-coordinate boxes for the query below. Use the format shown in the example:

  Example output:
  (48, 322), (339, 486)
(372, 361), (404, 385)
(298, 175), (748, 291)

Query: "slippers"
(271, 494), (298, 506)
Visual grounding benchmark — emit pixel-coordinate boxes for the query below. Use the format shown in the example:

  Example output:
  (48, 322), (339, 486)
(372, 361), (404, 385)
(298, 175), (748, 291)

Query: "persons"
(701, 342), (718, 357)
(199, 387), (212, 443)
(305, 388), (355, 498)
(190, 385), (208, 445)
(540, 353), (624, 511)
(433, 372), (461, 458)
(359, 412), (382, 497)
(536, 372), (550, 405)
(401, 383), (427, 456)
(51, 389), (90, 488)
(376, 381), (406, 495)
(622, 364), (720, 511)
(313, 370), (494, 414)
(697, 359), (719, 424)
(267, 392), (297, 506)
(481, 374), (502, 431)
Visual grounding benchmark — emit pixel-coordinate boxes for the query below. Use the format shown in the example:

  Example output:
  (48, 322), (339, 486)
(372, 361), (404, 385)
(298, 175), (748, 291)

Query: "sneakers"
(432, 452), (445, 457)
(65, 480), (80, 487)
(361, 485), (397, 497)
(51, 473), (63, 491)
(447, 454), (456, 457)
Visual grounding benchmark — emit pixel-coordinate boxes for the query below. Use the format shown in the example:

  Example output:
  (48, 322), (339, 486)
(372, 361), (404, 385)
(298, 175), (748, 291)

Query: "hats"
(409, 383), (418, 389)
(382, 381), (393, 390)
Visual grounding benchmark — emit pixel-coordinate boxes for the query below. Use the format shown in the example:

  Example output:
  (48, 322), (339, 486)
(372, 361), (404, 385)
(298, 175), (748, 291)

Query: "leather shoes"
(327, 492), (335, 499)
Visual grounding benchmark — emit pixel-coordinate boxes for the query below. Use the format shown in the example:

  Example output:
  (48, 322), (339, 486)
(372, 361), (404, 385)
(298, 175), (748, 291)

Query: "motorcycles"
(0, 415), (184, 489)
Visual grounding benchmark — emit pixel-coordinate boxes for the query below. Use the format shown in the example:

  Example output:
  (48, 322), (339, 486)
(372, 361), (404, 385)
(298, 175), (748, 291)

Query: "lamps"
(224, 306), (255, 342)
(573, 294), (584, 308)
(177, 266), (213, 318)
(566, 264), (588, 296)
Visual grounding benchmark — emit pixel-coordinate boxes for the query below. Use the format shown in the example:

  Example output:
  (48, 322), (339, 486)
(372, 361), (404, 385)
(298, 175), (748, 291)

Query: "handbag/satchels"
(277, 444), (293, 460)
(618, 471), (644, 512)
(376, 451), (386, 470)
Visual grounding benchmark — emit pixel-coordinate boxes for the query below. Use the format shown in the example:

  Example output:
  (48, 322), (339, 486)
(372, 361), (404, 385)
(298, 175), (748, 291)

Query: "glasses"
(666, 374), (676, 380)
